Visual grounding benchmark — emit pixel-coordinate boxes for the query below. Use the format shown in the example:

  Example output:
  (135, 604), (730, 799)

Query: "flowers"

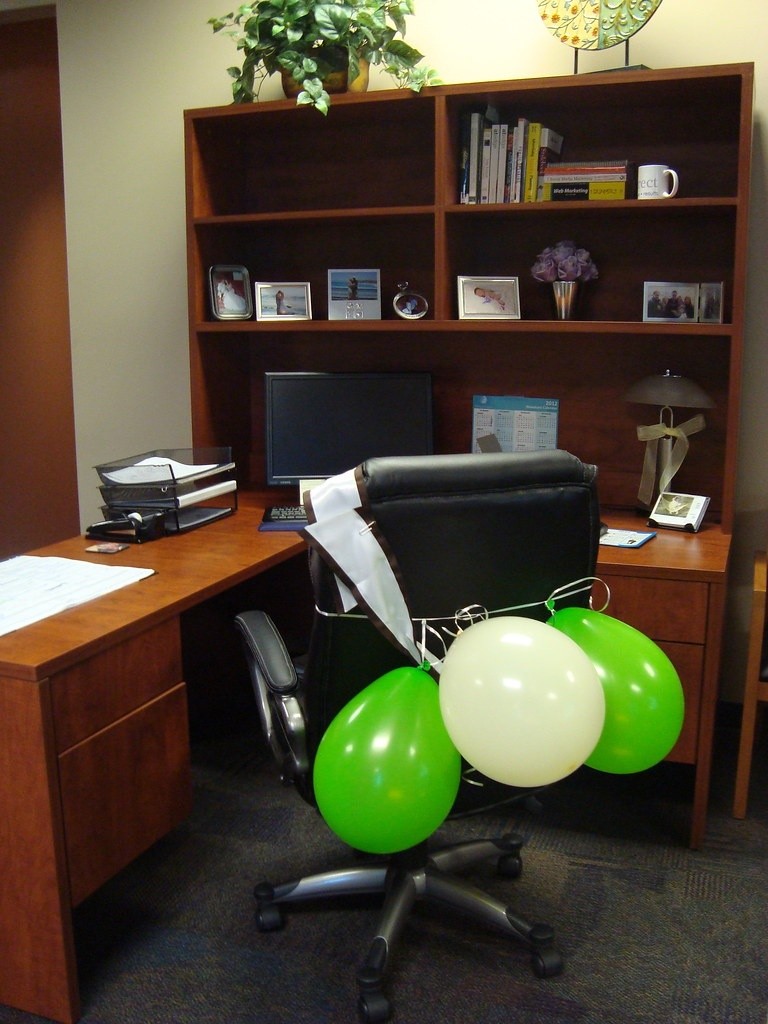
(532, 238), (600, 282)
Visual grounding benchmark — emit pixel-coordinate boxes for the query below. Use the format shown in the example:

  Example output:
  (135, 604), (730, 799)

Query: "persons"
(474, 288), (504, 310)
(648, 289), (695, 319)
(218, 280), (235, 310)
(347, 278), (358, 299)
(276, 291), (286, 315)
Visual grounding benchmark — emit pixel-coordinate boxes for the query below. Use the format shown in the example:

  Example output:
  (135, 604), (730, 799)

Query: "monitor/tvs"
(262, 370), (435, 487)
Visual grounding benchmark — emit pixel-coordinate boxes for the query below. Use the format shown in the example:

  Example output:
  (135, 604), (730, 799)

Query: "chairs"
(234, 450), (599, 1024)
(734, 551), (768, 820)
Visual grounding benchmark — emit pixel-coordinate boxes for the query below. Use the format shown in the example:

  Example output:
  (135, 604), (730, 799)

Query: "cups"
(637, 165), (679, 199)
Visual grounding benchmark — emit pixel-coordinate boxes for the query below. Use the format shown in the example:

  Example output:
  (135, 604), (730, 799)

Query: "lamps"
(621, 368), (717, 506)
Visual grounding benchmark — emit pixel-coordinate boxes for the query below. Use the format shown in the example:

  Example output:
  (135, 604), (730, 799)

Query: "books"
(459, 112), (635, 204)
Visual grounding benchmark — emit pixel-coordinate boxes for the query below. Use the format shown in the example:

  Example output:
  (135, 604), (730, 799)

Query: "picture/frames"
(642, 282), (700, 323)
(456, 276), (521, 319)
(255, 281), (313, 321)
(209, 265), (253, 321)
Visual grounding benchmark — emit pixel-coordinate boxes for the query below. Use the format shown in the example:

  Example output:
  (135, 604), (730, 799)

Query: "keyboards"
(258, 505), (310, 531)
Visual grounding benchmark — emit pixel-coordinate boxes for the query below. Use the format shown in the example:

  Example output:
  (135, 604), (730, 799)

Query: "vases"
(552, 280), (577, 321)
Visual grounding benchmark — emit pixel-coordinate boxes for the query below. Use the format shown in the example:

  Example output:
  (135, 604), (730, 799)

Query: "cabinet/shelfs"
(0, 508), (734, 1024)
(182, 61), (755, 536)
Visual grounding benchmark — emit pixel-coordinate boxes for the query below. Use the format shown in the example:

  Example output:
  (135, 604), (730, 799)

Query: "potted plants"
(207, 0), (443, 116)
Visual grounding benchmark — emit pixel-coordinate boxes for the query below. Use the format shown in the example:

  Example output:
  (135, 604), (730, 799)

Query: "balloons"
(438, 615), (605, 788)
(313, 661), (461, 855)
(545, 601), (685, 775)
